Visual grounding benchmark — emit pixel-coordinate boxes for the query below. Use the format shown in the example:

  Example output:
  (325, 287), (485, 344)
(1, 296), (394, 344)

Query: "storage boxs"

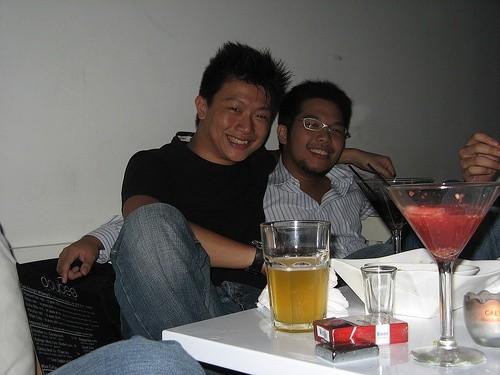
(313, 315), (408, 345)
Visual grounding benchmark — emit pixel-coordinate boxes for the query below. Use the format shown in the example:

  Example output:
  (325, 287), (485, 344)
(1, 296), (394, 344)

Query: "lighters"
(314, 338), (379, 363)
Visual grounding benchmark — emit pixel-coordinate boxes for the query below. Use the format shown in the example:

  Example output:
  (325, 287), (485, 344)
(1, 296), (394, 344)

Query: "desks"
(161, 286), (500, 375)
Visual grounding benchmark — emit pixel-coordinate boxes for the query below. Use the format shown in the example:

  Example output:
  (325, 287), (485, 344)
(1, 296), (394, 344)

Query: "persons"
(56, 80), (500, 288)
(49, 334), (209, 374)
(112, 42), (395, 341)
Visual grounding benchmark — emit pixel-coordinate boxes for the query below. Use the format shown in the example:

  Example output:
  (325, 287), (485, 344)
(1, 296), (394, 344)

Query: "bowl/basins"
(330, 247), (500, 319)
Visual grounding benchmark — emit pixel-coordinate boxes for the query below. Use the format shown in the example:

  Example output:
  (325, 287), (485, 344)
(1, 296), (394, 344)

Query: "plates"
(394, 263), (480, 276)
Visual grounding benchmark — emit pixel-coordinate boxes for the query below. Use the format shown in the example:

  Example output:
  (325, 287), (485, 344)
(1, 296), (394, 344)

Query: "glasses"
(291, 116), (346, 138)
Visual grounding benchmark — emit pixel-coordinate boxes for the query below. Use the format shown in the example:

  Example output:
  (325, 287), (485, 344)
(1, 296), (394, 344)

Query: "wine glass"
(384, 182), (500, 367)
(355, 178), (434, 254)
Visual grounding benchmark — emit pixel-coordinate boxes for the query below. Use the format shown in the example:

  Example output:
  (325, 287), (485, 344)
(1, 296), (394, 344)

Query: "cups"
(360, 265), (397, 325)
(261, 220), (332, 330)
(461, 291), (500, 348)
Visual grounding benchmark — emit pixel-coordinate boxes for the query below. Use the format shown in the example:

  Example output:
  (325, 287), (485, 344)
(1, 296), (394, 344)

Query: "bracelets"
(245, 242), (264, 274)
